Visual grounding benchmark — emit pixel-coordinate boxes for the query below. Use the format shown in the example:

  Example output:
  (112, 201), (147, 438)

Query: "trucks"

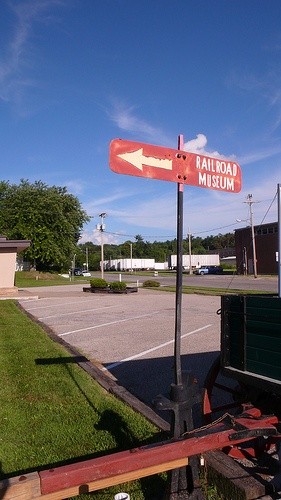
(196, 267), (223, 275)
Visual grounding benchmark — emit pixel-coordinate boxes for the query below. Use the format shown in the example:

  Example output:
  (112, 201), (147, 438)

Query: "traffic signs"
(108, 139), (241, 193)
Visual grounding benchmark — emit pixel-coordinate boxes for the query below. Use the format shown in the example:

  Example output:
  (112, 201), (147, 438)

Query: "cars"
(71, 269), (82, 276)
